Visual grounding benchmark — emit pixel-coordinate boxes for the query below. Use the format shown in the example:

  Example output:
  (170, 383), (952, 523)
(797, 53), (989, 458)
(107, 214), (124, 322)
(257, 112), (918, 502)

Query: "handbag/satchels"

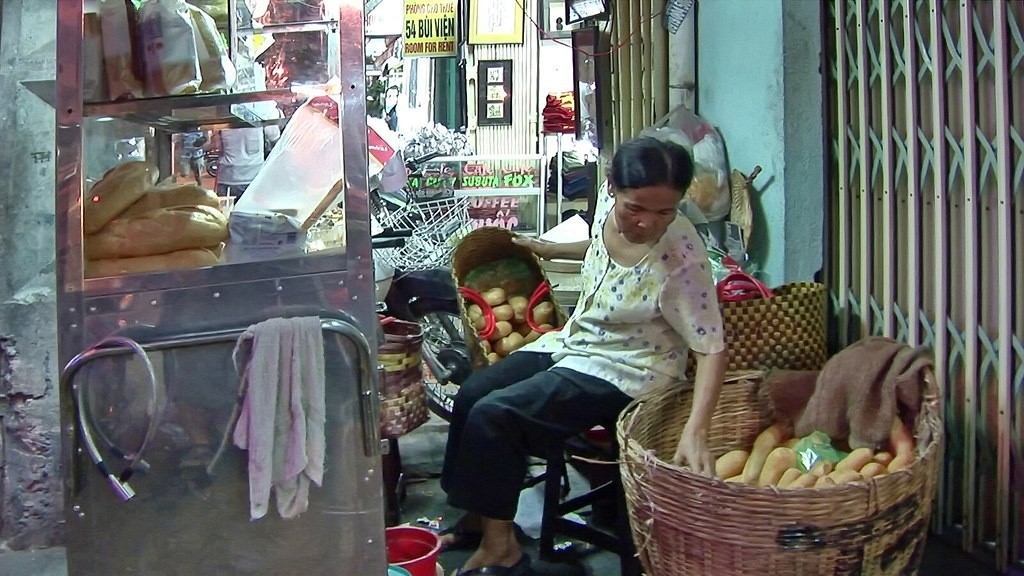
(693, 271), (828, 370)
(639, 103), (731, 224)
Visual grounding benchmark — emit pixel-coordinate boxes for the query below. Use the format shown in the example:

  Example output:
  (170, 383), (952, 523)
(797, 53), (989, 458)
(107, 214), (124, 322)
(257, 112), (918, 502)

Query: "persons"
(432, 137), (727, 576)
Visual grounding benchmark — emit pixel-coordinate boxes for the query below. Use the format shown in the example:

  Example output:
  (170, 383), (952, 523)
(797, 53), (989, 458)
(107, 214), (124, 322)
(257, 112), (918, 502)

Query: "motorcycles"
(372, 225), (476, 424)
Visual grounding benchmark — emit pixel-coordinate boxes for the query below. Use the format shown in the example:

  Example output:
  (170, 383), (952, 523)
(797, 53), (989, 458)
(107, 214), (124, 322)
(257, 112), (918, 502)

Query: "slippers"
(456, 551), (531, 576)
(436, 520), (521, 551)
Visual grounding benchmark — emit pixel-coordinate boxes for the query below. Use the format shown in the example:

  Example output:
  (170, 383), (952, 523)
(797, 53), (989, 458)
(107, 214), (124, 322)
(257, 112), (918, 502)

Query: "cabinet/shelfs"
(55, 0), (388, 576)
(404, 154), (546, 238)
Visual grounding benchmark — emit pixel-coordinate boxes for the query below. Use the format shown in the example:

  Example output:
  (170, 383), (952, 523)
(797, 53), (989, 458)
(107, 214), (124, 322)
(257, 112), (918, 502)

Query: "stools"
(540, 425), (646, 576)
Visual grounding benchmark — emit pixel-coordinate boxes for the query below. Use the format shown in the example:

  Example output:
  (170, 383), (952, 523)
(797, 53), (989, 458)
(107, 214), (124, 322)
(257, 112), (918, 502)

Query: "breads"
(467, 288), (555, 362)
(84, 161), (230, 280)
(706, 413), (911, 489)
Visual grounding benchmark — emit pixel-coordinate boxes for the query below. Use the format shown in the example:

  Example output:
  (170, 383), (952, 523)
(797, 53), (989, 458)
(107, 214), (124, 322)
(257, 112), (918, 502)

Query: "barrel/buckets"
(385, 526), (442, 576)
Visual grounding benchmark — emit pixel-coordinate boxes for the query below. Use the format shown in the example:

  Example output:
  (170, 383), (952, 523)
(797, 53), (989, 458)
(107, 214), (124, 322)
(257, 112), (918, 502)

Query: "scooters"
(206, 148), (219, 177)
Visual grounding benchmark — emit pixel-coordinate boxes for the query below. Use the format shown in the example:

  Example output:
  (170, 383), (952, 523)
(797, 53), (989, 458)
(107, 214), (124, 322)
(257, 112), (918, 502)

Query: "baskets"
(376, 311), (431, 439)
(451, 226), (569, 366)
